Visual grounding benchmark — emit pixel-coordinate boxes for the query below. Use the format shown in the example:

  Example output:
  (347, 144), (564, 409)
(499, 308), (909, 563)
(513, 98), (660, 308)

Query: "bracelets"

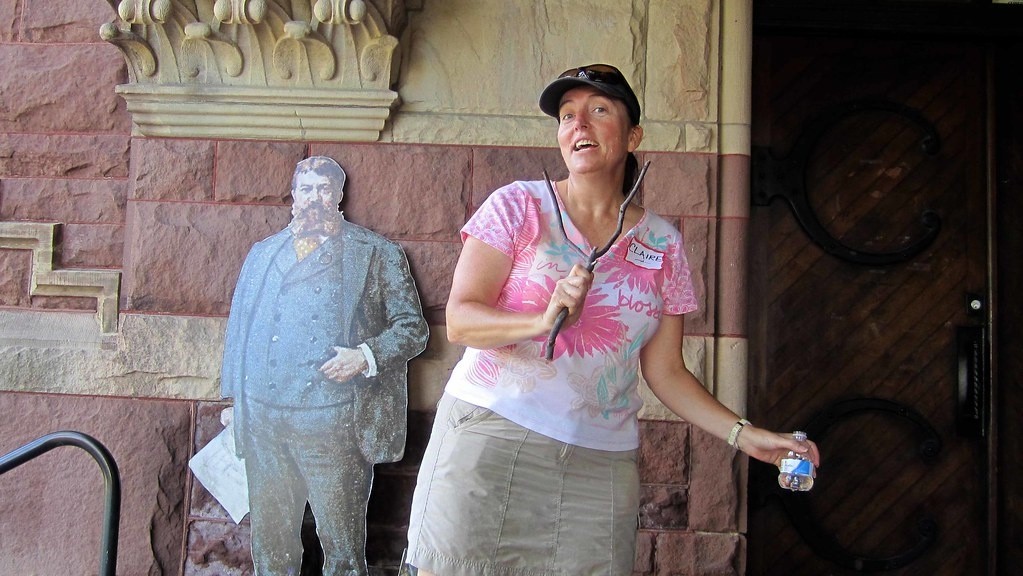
(727, 418), (752, 451)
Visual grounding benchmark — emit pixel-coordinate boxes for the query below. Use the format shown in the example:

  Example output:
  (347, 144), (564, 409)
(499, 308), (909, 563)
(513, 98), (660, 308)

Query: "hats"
(542, 67), (640, 126)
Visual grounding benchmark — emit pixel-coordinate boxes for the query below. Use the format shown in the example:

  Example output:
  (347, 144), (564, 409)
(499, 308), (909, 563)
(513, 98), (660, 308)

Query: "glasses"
(557, 64), (622, 86)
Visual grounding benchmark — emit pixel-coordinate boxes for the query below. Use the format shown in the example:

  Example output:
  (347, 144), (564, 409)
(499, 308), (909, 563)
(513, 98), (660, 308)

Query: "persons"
(219, 156), (429, 576)
(409, 62), (821, 576)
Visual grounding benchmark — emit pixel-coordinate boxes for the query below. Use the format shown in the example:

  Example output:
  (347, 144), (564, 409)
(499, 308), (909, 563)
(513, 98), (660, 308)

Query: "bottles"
(777, 430), (814, 492)
